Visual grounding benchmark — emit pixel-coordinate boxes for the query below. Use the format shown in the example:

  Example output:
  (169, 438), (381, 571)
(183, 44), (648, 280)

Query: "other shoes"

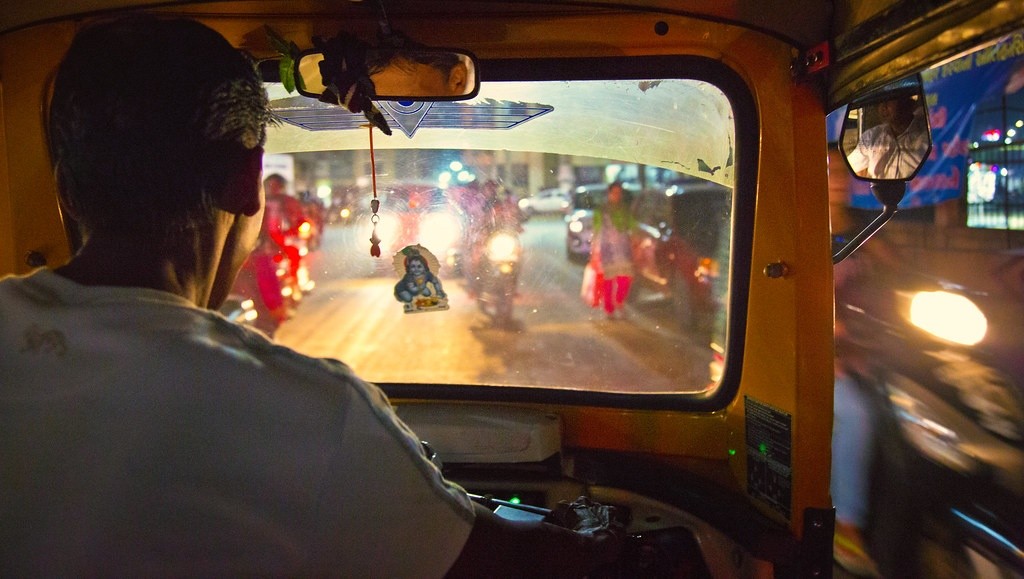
(616, 303), (626, 314)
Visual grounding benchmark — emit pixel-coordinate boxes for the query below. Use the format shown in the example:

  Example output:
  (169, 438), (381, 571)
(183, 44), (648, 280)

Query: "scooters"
(467, 225), (526, 325)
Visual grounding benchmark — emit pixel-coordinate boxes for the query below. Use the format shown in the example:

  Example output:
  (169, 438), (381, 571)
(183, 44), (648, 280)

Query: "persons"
(827, 141), (929, 579)
(362, 55), (469, 98)
(847, 95), (930, 179)
(969, 60), (1024, 154)
(457, 179), (527, 303)
(589, 180), (641, 320)
(0, 13), (698, 578)
(247, 173), (313, 339)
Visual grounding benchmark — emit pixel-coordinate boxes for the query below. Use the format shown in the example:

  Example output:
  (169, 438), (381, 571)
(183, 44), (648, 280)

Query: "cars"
(629, 183), (726, 327)
(517, 188), (573, 218)
(565, 184), (636, 262)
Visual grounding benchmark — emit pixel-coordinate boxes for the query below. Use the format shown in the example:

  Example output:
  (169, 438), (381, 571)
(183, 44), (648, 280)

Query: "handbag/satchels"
(580, 262), (604, 307)
